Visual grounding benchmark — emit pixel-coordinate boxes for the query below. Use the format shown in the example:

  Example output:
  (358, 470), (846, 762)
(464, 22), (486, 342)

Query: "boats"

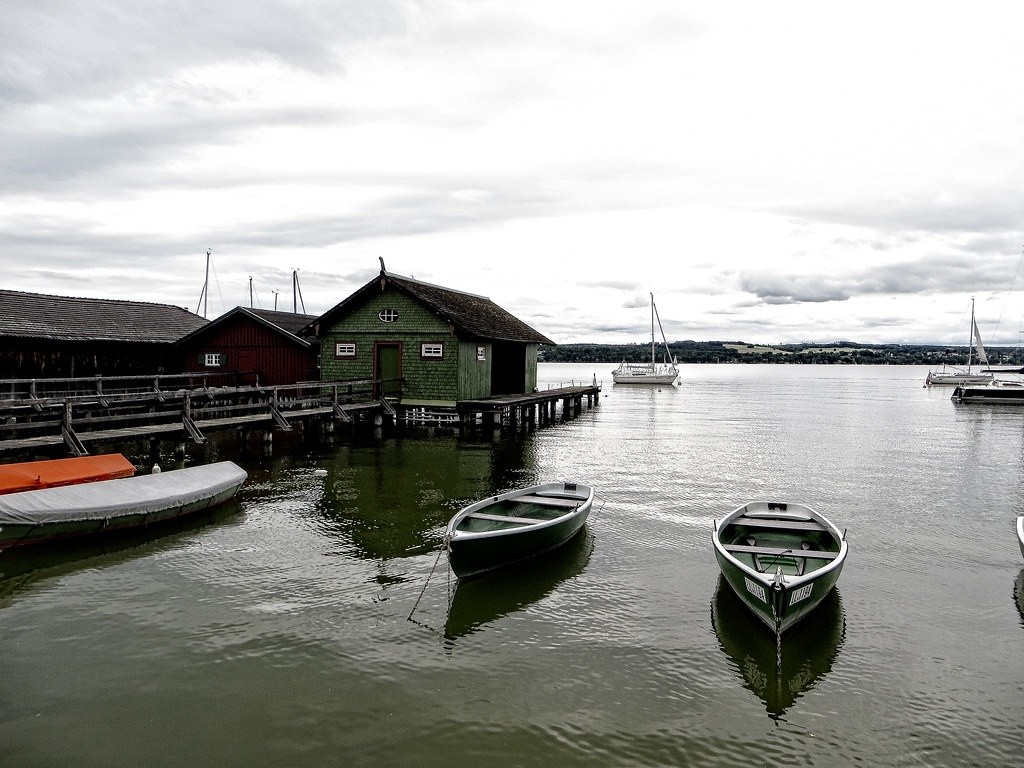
(711, 500), (848, 634)
(444, 523), (595, 661)
(442, 480), (594, 578)
(0, 495), (247, 611)
(712, 572), (846, 721)
(951, 385), (1024, 404)
(0, 460), (248, 547)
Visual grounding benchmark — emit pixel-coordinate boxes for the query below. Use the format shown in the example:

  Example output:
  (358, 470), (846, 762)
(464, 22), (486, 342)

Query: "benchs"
(722, 543), (839, 561)
(466, 511), (548, 526)
(730, 516), (827, 532)
(511, 495), (585, 509)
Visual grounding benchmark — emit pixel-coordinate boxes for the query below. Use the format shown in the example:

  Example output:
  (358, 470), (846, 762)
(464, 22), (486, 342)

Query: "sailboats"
(980, 250), (1024, 373)
(927, 297), (994, 386)
(613, 292), (678, 385)
